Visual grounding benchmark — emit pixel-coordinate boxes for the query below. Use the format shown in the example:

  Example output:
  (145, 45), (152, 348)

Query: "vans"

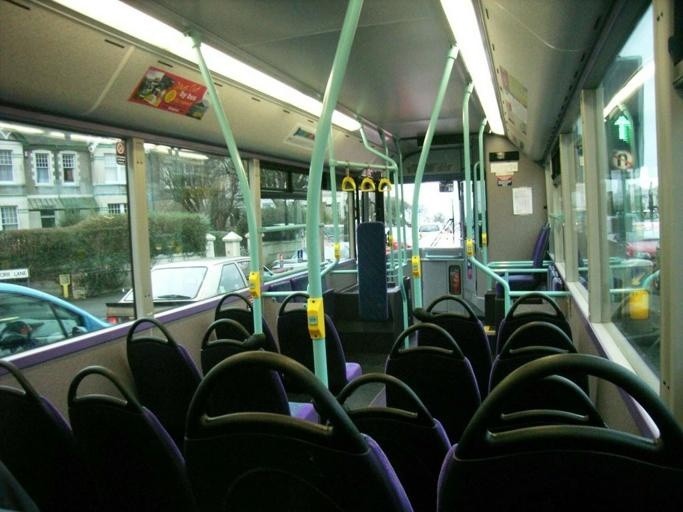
(415, 223), (453, 247)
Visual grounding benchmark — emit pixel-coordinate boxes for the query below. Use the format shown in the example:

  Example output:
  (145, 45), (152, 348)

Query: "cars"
(0, 278), (111, 357)
(103, 256), (273, 324)
(575, 208), (661, 260)
(260, 224), (350, 283)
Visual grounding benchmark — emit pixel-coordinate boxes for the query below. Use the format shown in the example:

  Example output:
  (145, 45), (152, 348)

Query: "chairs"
(265, 272), (337, 324)
(495, 215), (550, 293)
